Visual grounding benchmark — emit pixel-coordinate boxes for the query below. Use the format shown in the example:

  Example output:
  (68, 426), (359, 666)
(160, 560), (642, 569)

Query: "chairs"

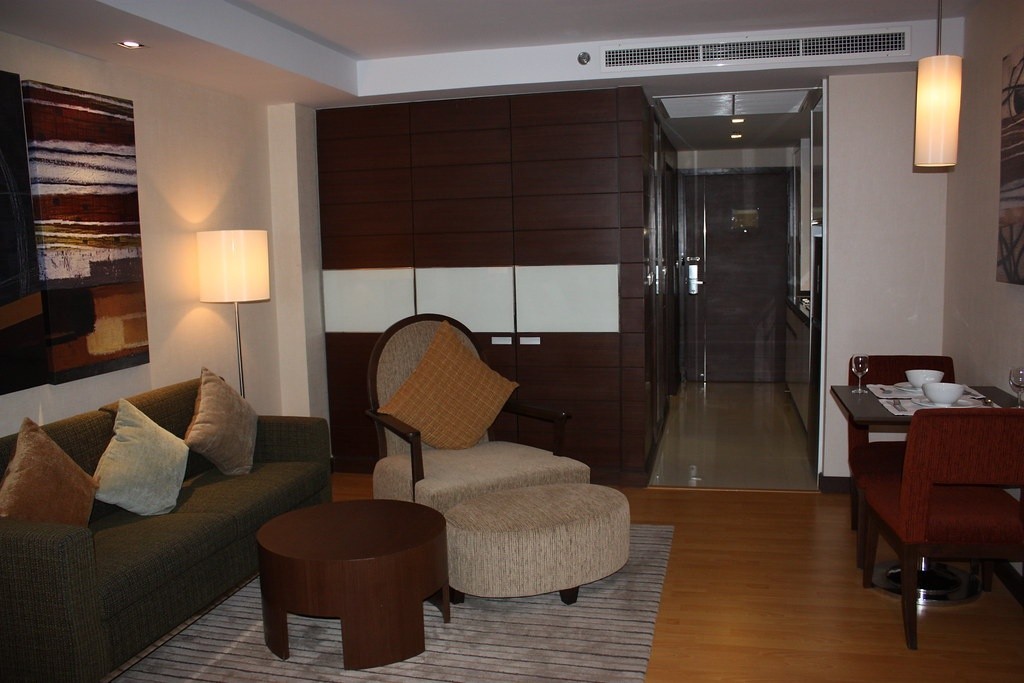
(366, 312), (591, 514)
(864, 408), (1024, 650)
(848, 354), (956, 568)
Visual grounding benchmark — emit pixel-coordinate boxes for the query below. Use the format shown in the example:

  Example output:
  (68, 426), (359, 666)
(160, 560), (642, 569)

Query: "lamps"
(914, 0), (962, 167)
(197, 229), (270, 399)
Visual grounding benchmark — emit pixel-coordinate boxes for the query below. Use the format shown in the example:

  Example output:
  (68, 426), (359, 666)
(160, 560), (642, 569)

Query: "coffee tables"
(256, 499), (450, 670)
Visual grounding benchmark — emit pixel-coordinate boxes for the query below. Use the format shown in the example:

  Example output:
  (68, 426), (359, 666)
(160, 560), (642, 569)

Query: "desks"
(830, 385), (1024, 423)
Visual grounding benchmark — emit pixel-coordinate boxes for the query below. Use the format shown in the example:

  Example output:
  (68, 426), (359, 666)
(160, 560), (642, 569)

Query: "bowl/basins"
(923, 382), (965, 408)
(906, 369), (944, 390)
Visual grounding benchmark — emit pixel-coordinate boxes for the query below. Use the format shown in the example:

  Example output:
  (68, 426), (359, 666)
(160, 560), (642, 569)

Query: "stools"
(445, 483), (631, 606)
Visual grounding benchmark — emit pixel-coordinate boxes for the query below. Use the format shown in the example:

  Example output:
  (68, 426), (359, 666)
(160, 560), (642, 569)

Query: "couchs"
(0, 382), (331, 683)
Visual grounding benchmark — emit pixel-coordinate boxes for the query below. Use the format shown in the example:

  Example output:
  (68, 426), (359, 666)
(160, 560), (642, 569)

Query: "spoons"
(880, 387), (892, 394)
(982, 398), (996, 408)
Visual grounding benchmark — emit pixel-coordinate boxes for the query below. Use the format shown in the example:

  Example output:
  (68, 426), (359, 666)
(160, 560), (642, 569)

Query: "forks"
(894, 398), (908, 411)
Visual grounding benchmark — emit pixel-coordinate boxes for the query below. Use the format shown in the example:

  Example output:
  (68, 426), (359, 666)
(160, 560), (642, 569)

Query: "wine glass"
(851, 353), (870, 394)
(1008, 368), (1024, 409)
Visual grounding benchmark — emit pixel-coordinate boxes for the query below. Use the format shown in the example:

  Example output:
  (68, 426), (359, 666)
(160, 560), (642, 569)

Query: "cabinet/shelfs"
(314, 86), (656, 486)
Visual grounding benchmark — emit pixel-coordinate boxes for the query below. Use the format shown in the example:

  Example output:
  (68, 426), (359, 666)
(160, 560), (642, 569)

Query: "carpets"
(106, 525), (675, 682)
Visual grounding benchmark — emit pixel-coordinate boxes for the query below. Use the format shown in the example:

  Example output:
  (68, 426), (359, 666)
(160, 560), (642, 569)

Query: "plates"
(911, 394), (983, 408)
(894, 382), (923, 392)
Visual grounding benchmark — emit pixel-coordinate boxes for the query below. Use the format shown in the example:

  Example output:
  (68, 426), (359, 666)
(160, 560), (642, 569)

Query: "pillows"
(183, 366), (258, 475)
(378, 320), (520, 450)
(93, 398), (188, 517)
(0, 416), (101, 528)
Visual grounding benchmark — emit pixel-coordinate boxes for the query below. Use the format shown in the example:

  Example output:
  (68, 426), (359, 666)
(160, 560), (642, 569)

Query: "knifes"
(886, 400), (901, 411)
